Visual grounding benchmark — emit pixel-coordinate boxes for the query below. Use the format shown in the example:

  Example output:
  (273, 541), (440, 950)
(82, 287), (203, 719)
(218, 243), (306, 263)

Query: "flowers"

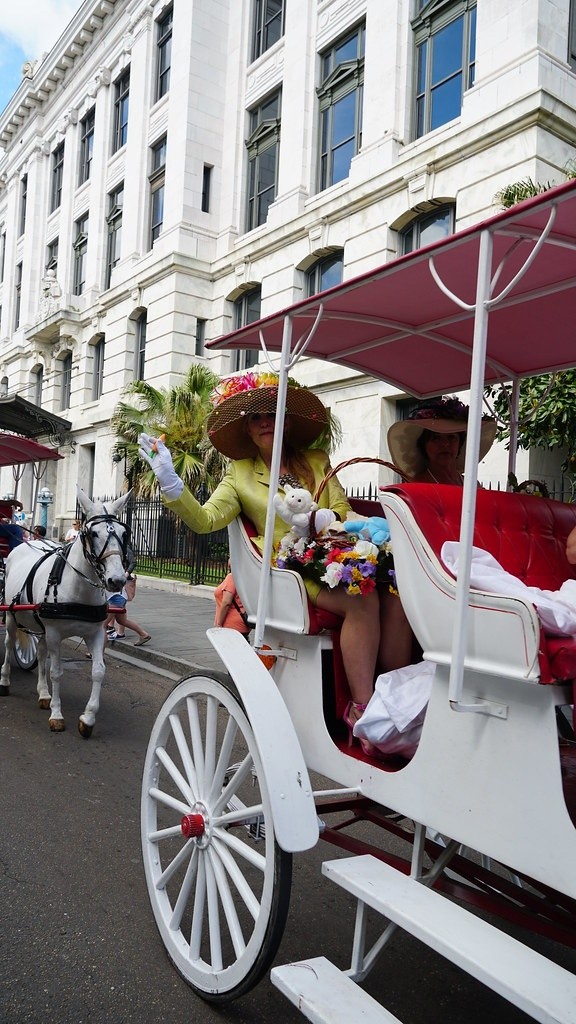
(270, 535), (402, 597)
(209, 371), (279, 406)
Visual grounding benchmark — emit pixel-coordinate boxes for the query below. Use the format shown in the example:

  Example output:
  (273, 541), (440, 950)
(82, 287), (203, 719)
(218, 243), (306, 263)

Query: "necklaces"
(427, 469), (463, 484)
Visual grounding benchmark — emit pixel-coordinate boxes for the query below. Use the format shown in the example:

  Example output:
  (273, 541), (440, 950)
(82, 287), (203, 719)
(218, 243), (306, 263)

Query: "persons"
(387, 399), (497, 488)
(138, 373), (412, 754)
(106, 548), (137, 640)
(85, 585), (151, 658)
(65, 520), (81, 542)
(33, 526), (47, 540)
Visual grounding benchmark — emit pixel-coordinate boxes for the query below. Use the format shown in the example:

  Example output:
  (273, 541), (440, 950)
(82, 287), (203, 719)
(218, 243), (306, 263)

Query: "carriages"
(140, 175), (576, 1024)
(0, 432), (133, 738)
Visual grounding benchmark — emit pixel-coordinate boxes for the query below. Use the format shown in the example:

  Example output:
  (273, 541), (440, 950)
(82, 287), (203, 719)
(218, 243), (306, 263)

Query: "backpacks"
(232, 596), (256, 629)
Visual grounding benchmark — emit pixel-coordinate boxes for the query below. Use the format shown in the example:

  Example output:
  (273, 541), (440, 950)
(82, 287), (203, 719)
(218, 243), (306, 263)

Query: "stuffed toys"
(274, 485), (318, 550)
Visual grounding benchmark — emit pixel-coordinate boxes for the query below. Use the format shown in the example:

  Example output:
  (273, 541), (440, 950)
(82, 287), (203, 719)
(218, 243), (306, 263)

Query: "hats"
(206, 371), (328, 460)
(387, 396), (497, 480)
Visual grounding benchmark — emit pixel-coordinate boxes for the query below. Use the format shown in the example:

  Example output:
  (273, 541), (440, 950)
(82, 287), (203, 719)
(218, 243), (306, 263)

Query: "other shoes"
(117, 634), (126, 639)
(134, 636), (151, 647)
(106, 627), (115, 633)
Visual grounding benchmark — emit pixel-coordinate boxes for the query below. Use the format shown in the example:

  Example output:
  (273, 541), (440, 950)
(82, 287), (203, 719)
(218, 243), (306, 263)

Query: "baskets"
(309, 457), (415, 582)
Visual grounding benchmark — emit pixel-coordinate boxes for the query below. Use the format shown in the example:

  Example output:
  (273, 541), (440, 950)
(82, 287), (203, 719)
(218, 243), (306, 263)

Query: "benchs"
(0, 506), (12, 557)
(227, 497), (385, 637)
(377, 481), (576, 687)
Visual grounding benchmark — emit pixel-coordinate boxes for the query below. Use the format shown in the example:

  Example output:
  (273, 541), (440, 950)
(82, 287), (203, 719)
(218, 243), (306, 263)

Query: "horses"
(0, 484), (135, 740)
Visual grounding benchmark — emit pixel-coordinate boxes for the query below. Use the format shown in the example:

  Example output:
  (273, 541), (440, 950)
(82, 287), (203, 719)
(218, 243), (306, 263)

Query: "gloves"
(290, 508), (336, 537)
(137, 433), (183, 503)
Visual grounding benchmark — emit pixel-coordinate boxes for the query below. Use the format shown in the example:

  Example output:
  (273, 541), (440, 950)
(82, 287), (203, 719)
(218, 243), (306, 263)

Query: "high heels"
(344, 701), (392, 759)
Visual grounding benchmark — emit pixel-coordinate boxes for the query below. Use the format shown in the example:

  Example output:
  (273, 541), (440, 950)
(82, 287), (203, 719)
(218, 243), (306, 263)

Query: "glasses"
(73, 524), (76, 525)
